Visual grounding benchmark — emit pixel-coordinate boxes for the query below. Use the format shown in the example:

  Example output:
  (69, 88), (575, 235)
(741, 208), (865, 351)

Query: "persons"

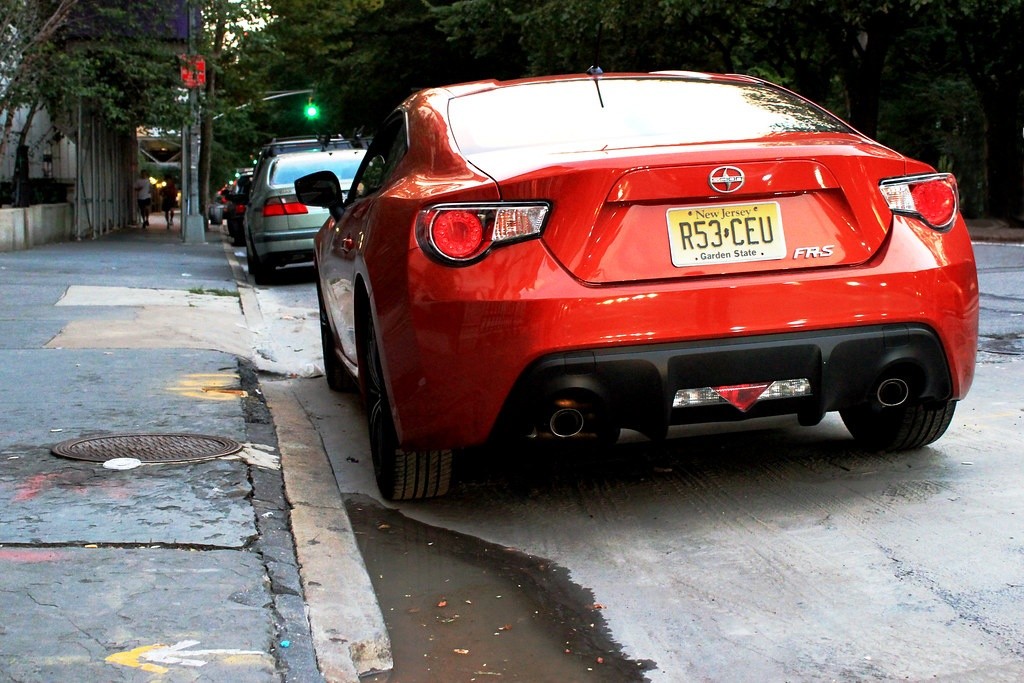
(159, 174), (178, 229)
(134, 170), (152, 228)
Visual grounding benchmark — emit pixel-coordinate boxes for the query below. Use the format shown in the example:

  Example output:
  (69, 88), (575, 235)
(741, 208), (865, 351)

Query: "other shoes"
(167, 222), (169, 229)
(169, 219), (172, 225)
(143, 221), (146, 228)
(146, 220), (149, 226)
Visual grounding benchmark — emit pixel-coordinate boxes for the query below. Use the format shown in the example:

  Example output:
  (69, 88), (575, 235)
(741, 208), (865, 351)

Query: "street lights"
(186, 90), (320, 216)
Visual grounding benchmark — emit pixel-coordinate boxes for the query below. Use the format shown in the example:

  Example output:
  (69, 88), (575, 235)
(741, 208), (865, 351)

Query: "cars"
(229, 149), (367, 285)
(216, 185), (235, 219)
(295, 69), (979, 501)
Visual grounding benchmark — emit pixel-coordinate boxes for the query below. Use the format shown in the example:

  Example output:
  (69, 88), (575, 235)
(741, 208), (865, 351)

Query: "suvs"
(237, 134), (371, 200)
(227, 171), (254, 245)
